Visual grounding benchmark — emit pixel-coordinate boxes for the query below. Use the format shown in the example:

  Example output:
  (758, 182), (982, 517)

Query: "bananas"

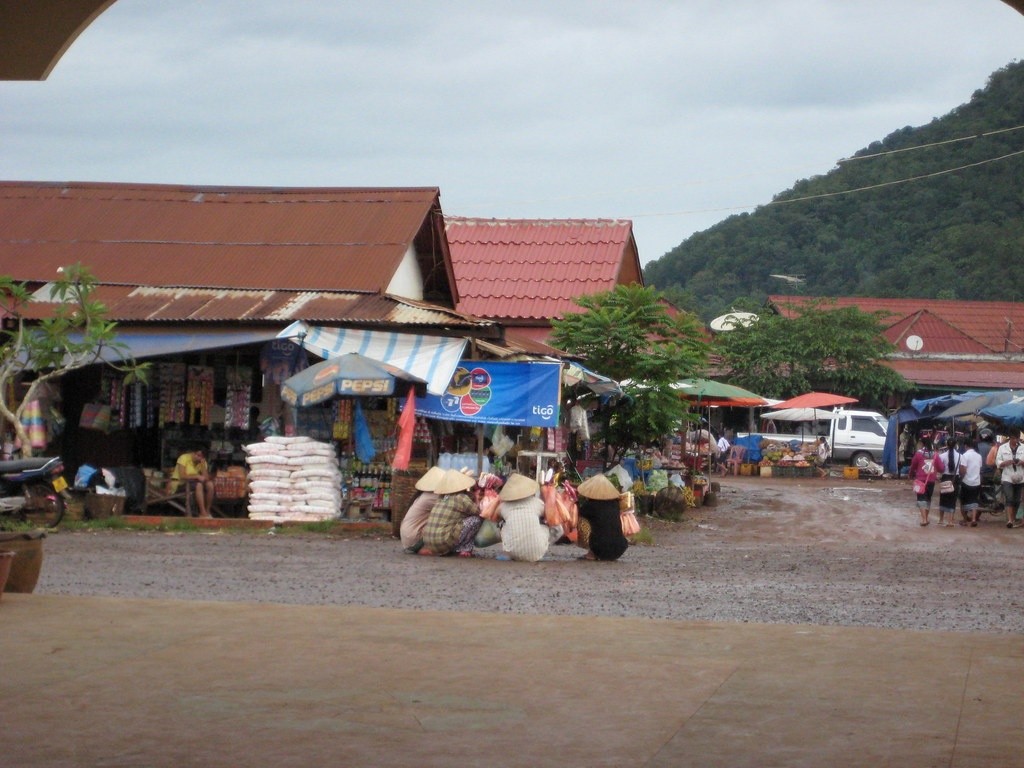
(637, 459), (652, 470)
(681, 486), (696, 506)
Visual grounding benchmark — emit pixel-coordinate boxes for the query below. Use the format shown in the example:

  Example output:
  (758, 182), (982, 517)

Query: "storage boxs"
(741, 464), (757, 475)
(759, 466), (816, 477)
(843, 466), (858, 479)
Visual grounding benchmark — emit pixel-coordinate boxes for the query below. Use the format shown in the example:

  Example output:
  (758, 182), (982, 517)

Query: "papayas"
(763, 452), (783, 461)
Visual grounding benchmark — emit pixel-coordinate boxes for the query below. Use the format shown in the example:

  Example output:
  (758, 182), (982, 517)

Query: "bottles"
(352, 462), (391, 488)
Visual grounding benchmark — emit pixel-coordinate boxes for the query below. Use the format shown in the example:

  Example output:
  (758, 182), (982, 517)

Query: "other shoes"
(946, 523), (955, 527)
(919, 521), (930, 526)
(722, 470), (729, 478)
(971, 521), (977, 526)
(459, 552), (475, 557)
(1012, 522), (1018, 528)
(1006, 522), (1013, 528)
(199, 514), (213, 519)
(937, 520), (944, 524)
(417, 548), (434, 555)
(959, 519), (967, 526)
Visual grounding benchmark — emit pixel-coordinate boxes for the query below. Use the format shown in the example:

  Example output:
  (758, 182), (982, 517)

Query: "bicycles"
(961, 469), (1006, 522)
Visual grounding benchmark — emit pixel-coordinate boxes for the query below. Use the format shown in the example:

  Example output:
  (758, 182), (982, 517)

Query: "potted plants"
(0, 515), (48, 594)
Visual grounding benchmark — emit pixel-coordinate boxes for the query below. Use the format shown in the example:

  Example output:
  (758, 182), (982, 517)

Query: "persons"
(167, 446), (213, 519)
(498, 474), (549, 562)
(399, 466), (481, 557)
(715, 433), (730, 477)
(814, 437), (831, 478)
(909, 429), (1024, 527)
(575, 473), (631, 562)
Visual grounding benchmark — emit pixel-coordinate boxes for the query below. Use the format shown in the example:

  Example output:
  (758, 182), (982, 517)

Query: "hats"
(433, 468), (476, 494)
(499, 473), (538, 501)
(414, 466), (445, 492)
(577, 473), (620, 501)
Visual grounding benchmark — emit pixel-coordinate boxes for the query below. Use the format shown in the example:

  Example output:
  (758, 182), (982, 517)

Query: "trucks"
(737, 406), (889, 473)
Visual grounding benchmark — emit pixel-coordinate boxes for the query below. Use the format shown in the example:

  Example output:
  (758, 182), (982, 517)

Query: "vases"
(1, 552), (16, 593)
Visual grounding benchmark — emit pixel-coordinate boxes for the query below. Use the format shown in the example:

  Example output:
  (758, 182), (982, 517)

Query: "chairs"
(726, 446), (746, 476)
(67, 454), (196, 517)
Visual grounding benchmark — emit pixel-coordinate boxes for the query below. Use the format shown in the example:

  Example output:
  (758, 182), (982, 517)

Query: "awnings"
(541, 355), (626, 404)
(277, 320), (468, 396)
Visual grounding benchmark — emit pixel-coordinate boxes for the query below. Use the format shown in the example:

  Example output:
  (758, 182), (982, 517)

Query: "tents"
(882, 404), (971, 475)
(911, 391), (1024, 426)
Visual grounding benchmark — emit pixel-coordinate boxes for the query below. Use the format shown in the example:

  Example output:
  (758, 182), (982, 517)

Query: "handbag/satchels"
(912, 479), (926, 494)
(939, 481), (954, 493)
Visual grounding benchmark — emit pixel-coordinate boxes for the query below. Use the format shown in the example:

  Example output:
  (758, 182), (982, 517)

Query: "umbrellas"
(570, 373), (859, 505)
(280, 351), (429, 522)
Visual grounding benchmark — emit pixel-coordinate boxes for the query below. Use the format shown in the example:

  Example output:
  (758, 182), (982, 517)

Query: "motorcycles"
(0, 456), (68, 529)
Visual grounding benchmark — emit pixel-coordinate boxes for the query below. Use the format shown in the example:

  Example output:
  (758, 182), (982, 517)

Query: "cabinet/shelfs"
(148, 439), (247, 487)
